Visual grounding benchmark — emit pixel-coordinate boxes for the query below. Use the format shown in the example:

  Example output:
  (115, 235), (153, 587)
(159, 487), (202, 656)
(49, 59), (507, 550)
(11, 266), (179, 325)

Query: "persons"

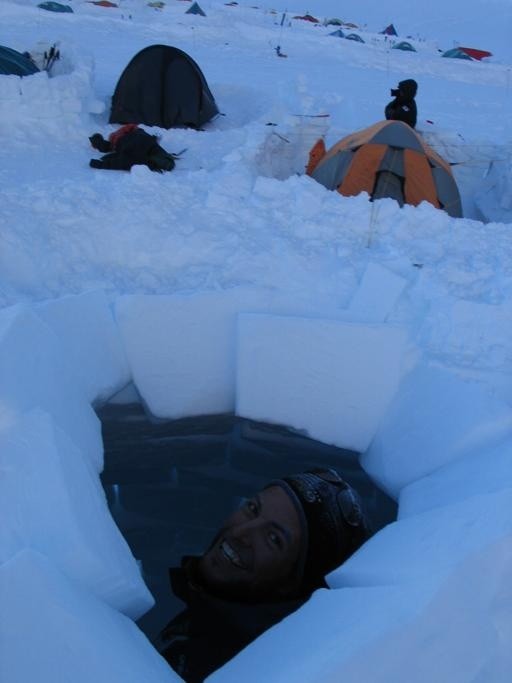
(383, 77), (417, 129)
(158, 466), (373, 681)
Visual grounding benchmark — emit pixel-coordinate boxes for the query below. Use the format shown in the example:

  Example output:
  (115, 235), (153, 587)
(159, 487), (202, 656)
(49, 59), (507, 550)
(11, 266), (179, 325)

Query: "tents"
(107, 41), (226, 129)
(378, 22), (397, 35)
(324, 16), (346, 26)
(184, 1), (204, 14)
(329, 27), (347, 38)
(304, 117), (465, 217)
(291, 12), (319, 22)
(1, 43), (41, 76)
(391, 40), (416, 51)
(347, 31), (366, 43)
(442, 45), (491, 60)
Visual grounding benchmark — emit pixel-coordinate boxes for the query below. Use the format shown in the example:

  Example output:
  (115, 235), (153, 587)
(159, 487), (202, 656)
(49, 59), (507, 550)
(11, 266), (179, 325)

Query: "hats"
(264, 469), (360, 575)
